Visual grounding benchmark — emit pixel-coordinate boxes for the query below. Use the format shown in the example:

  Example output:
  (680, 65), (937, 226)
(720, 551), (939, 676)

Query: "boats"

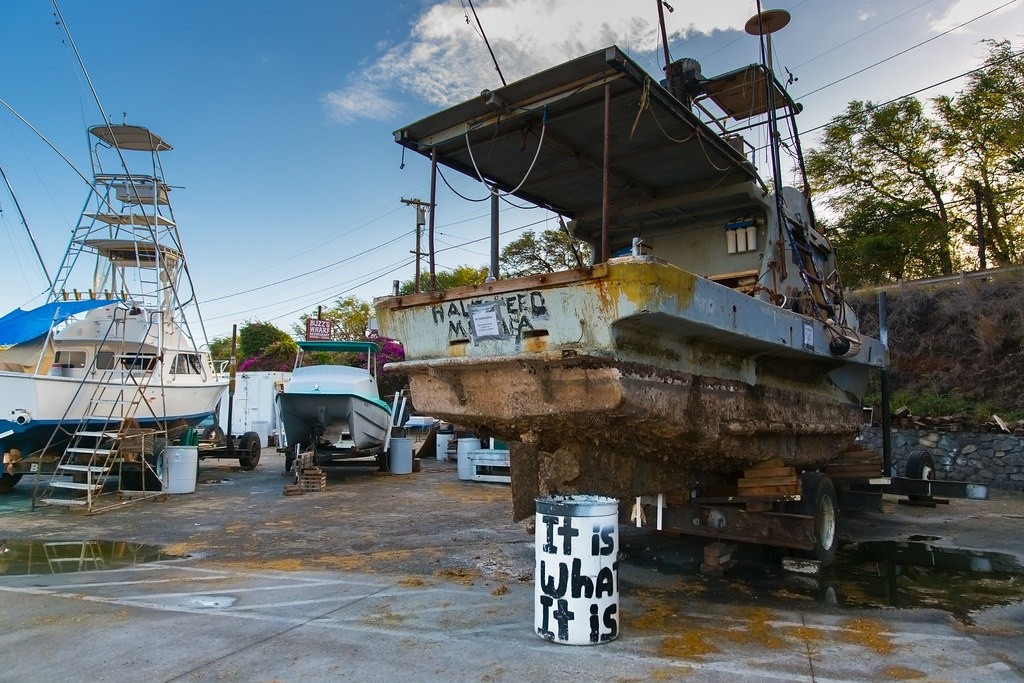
(276, 339), (392, 464)
(0, 124), (228, 475)
(369, 4), (892, 469)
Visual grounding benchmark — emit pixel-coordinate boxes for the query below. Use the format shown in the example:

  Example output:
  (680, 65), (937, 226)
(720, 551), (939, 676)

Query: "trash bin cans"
(163, 445), (199, 494)
(436, 430), (455, 460)
(458, 436), (482, 480)
(390, 437), (414, 476)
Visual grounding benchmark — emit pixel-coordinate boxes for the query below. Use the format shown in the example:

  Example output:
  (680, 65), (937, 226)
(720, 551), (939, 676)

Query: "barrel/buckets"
(457, 438), (481, 480)
(390, 437), (412, 474)
(161, 446), (198, 493)
(436, 430), (454, 461)
(49, 363), (63, 376)
(534, 494), (620, 646)
